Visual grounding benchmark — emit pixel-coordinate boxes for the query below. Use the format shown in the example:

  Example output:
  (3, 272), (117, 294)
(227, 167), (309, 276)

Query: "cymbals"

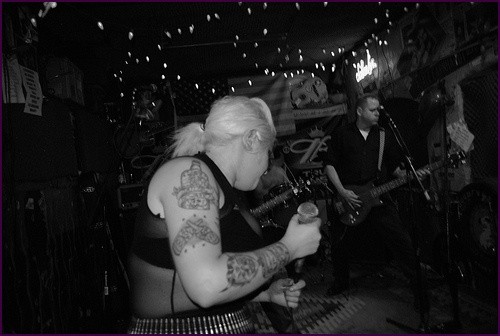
(292, 163), (323, 169)
(146, 123), (175, 137)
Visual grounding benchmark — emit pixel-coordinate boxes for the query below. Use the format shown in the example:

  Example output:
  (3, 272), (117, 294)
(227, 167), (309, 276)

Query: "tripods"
(385, 123), (451, 334)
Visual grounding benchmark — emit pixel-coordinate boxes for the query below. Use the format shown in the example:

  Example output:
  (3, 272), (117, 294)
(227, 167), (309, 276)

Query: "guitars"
(250, 174), (329, 217)
(334, 151), (467, 225)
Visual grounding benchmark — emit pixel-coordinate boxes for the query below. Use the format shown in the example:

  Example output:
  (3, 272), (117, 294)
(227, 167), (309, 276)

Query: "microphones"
(294, 202), (319, 284)
(380, 105), (397, 128)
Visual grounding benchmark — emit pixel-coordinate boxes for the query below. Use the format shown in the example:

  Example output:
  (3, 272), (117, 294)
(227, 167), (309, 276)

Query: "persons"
(325, 93), (447, 292)
(126, 95), (322, 334)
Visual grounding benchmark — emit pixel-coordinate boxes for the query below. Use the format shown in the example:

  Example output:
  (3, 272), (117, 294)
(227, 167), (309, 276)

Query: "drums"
(264, 182), (301, 226)
(371, 97), (432, 160)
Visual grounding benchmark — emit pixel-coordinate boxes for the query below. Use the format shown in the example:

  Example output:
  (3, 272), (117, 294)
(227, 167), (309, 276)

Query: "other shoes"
(410, 281), (432, 314)
(326, 277), (353, 296)
